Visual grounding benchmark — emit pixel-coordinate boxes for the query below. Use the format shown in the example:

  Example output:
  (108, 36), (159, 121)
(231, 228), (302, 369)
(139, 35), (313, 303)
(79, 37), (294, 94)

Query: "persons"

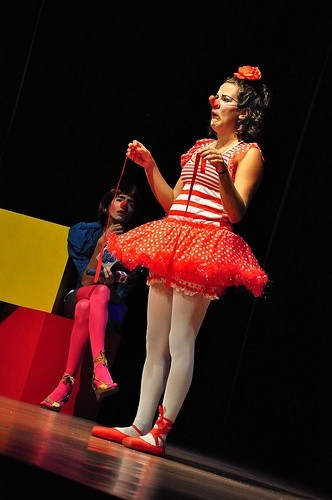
(39, 186), (137, 411)
(91, 66), (269, 458)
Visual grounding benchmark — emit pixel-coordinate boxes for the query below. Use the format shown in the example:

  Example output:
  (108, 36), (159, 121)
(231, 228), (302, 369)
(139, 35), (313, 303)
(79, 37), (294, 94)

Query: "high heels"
(40, 372), (75, 412)
(92, 350), (120, 402)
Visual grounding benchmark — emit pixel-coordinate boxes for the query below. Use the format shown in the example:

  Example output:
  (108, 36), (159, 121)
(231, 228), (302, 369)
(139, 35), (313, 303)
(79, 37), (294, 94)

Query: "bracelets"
(217, 169), (228, 174)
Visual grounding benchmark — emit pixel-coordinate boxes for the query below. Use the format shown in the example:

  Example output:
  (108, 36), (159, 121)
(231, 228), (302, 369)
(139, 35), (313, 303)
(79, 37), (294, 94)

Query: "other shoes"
(122, 406), (174, 457)
(91, 425), (143, 444)
